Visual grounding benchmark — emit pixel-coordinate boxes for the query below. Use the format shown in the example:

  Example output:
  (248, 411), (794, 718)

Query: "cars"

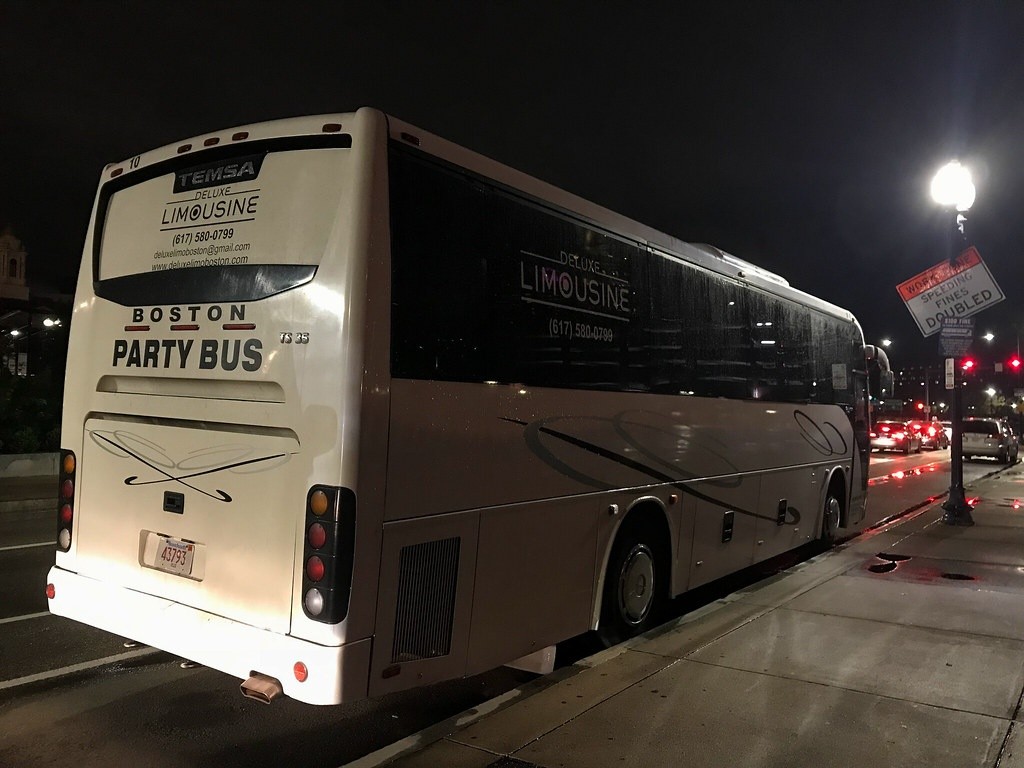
(907, 419), (950, 449)
(960, 416), (1018, 465)
(870, 420), (922, 454)
(930, 421), (952, 443)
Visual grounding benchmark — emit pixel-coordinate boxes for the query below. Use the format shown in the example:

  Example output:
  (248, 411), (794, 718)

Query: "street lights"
(928, 161), (978, 527)
(880, 337), (893, 349)
(979, 333), (995, 342)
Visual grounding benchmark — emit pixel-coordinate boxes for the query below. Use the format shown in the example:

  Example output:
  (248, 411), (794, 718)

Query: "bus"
(44, 107), (896, 707)
